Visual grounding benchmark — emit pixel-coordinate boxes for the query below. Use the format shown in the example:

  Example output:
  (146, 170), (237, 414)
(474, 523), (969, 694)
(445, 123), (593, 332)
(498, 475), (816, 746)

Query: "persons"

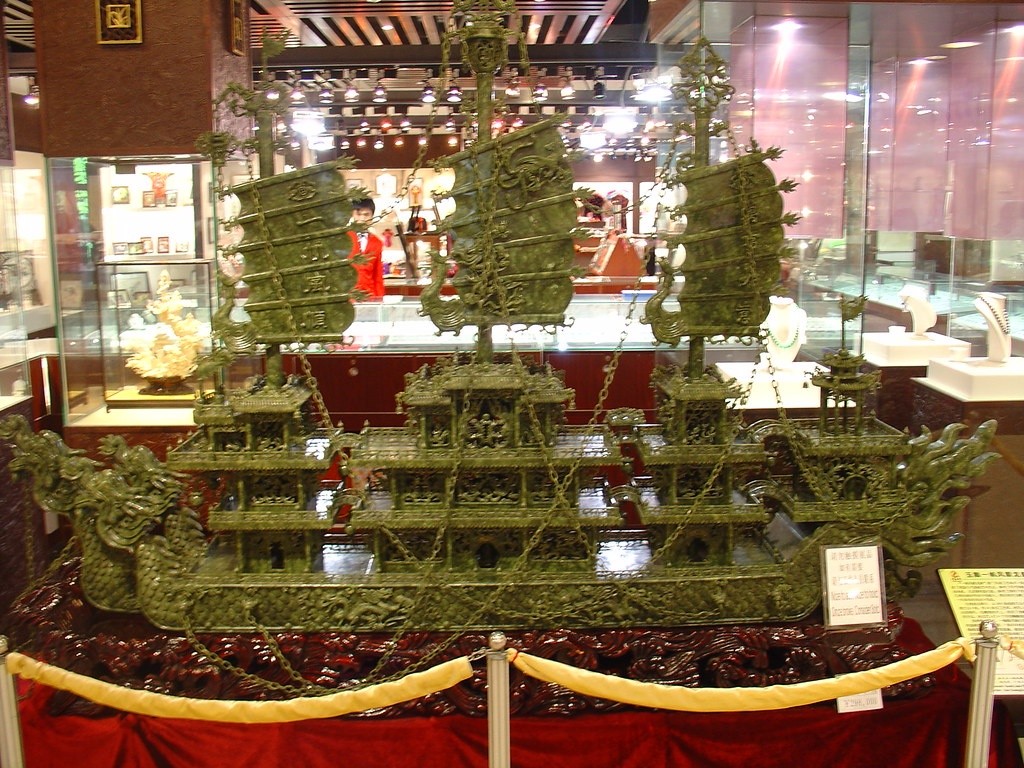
(347, 196), (385, 302)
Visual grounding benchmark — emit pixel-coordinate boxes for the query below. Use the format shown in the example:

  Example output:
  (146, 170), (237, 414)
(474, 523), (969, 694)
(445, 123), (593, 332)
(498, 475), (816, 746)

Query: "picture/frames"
(112, 186), (130, 204)
(112, 241), (129, 255)
(142, 190), (156, 207)
(95, 0), (143, 45)
(140, 236), (154, 254)
(157, 236), (169, 254)
(230, 0), (246, 57)
(407, 178), (423, 208)
(175, 241), (190, 253)
(128, 241), (147, 255)
(157, 279), (185, 290)
(133, 291), (152, 308)
(109, 271), (150, 301)
(346, 178), (362, 190)
(106, 288), (132, 309)
(164, 189), (178, 207)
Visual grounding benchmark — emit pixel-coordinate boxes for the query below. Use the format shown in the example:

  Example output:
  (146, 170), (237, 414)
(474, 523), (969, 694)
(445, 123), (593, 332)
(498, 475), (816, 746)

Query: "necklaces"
(767, 323), (802, 348)
(979, 295), (1011, 335)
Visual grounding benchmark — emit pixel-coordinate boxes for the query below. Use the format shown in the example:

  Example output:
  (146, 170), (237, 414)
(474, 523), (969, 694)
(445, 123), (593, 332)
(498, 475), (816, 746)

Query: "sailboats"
(0, 0), (1003, 628)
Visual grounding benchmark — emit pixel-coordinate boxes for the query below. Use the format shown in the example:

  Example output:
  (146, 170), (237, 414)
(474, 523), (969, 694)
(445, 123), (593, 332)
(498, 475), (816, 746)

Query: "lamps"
(560, 84), (576, 100)
(373, 138), (384, 149)
(358, 119), (370, 132)
(372, 87), (388, 103)
(446, 85), (462, 103)
(289, 89), (305, 104)
(532, 86), (549, 102)
(380, 119), (392, 131)
(339, 138), (350, 150)
(356, 137), (367, 148)
(421, 87), (436, 103)
(267, 88), (280, 100)
(318, 90), (334, 103)
(506, 77), (521, 95)
(445, 119), (456, 131)
(594, 82), (607, 100)
(447, 136), (458, 149)
(463, 119), (653, 162)
(393, 135), (405, 148)
(344, 89), (360, 102)
(399, 119), (413, 131)
